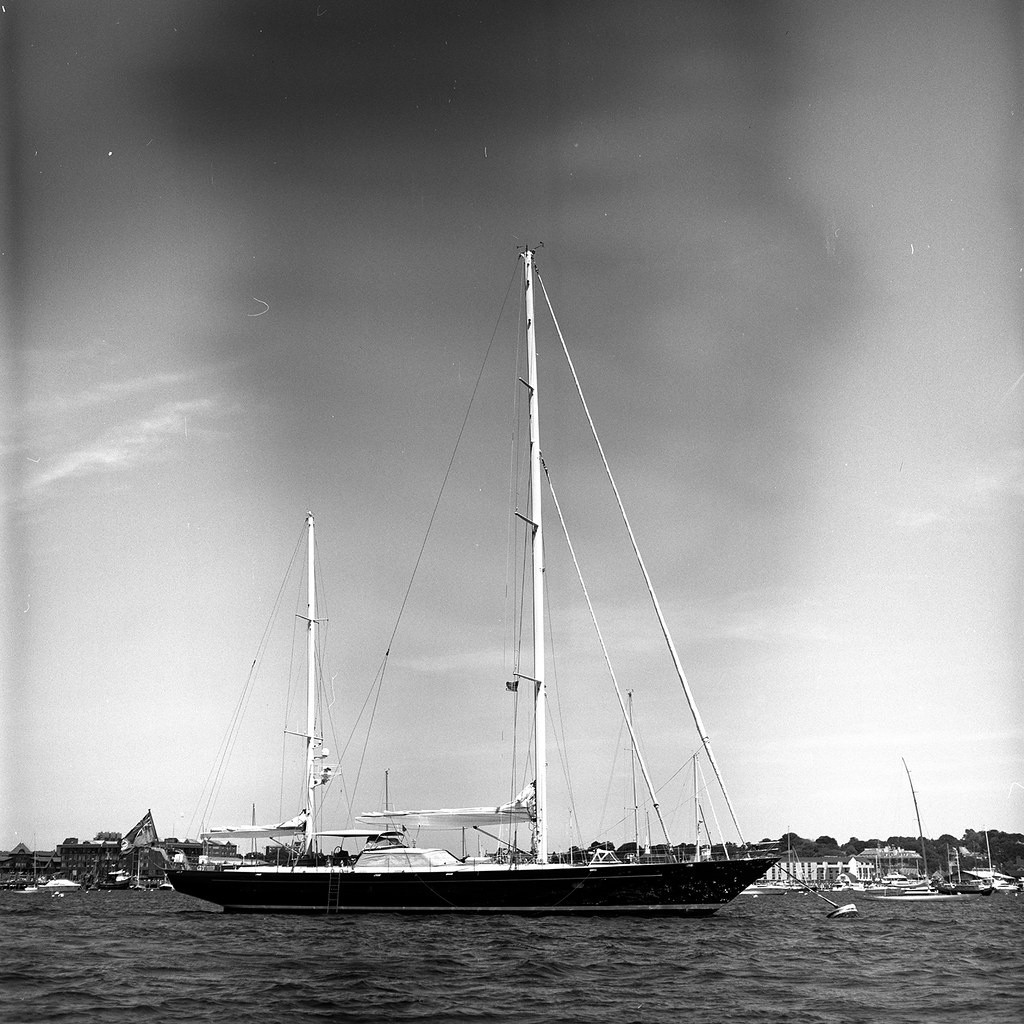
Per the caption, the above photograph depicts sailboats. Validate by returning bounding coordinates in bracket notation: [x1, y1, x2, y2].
[154, 246, 846, 915]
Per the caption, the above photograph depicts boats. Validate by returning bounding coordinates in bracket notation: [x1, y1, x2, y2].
[11, 830, 134, 897]
[857, 764, 1021, 903]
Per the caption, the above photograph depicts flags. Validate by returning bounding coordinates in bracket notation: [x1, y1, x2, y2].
[116, 812, 160, 860]
